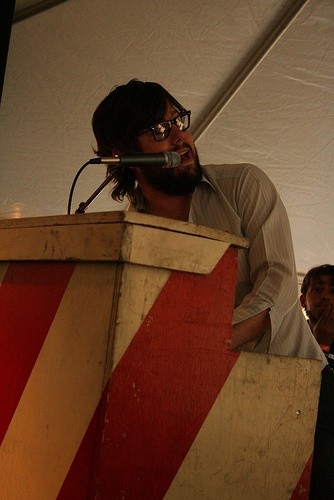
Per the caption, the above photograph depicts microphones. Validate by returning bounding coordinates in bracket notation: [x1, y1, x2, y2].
[89, 150, 181, 169]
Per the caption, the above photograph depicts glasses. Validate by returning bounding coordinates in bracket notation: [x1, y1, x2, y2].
[139, 110, 191, 142]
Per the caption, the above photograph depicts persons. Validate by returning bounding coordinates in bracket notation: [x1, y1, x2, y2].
[90, 79, 334, 500]
